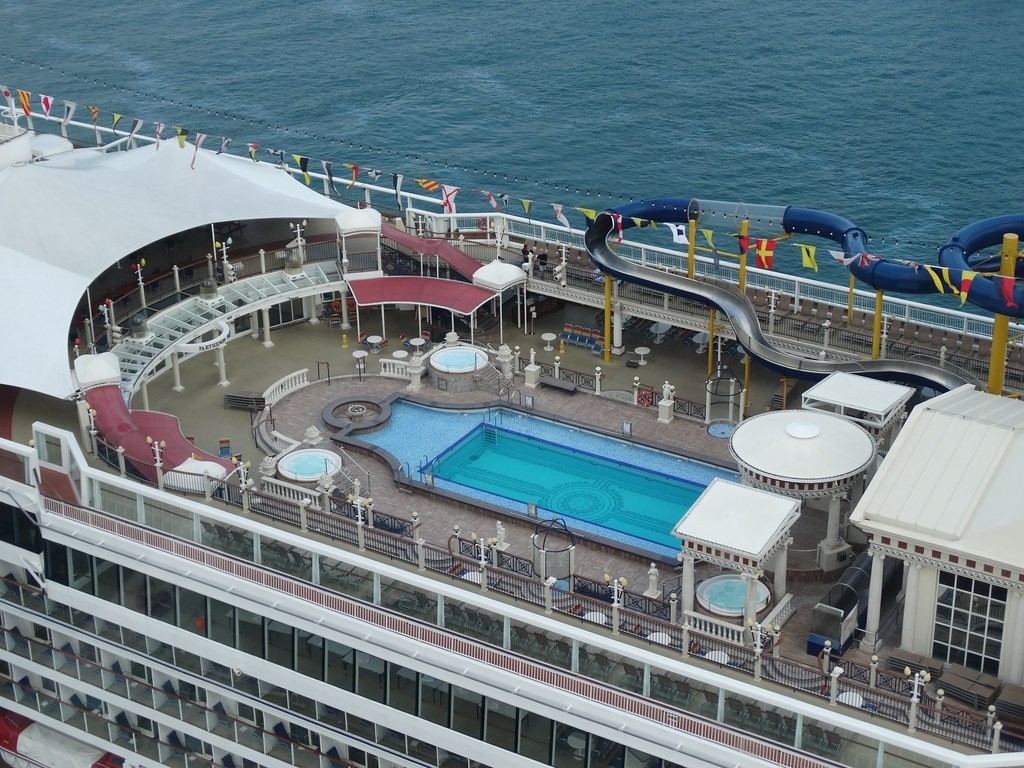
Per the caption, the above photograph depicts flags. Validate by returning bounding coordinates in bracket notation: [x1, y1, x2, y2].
[88, 106, 99, 126]
[321, 161, 340, 195]
[0, 85, 11, 100]
[248, 143, 260, 162]
[703, 228, 818, 271]
[666, 223, 689, 244]
[216, 137, 231, 155]
[830, 249, 1018, 308]
[367, 167, 403, 212]
[176, 128, 188, 148]
[577, 207, 623, 245]
[418, 179, 438, 192]
[191, 133, 205, 169]
[154, 123, 165, 151]
[474, 189, 572, 235]
[64, 101, 76, 125]
[632, 217, 656, 229]
[344, 163, 358, 190]
[17, 90, 32, 116]
[112, 114, 121, 134]
[267, 148, 310, 185]
[443, 186, 459, 213]
[40, 95, 54, 120]
[126, 119, 143, 151]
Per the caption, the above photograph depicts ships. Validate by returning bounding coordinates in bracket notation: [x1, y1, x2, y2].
[0, 107, 1024, 768]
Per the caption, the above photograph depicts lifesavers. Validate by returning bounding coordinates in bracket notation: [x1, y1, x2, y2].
[637, 391, 652, 408]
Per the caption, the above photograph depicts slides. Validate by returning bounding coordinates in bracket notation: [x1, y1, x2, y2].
[584, 197, 1024, 394]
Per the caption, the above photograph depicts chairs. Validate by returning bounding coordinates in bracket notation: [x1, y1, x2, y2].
[675, 639, 707, 656]
[185, 436, 195, 446]
[359, 719, 479, 768]
[885, 320, 1024, 382]
[513, 238, 598, 282]
[557, 642, 854, 768]
[360, 332, 388, 353]
[619, 618, 648, 637]
[400, 331, 430, 353]
[558, 309, 739, 358]
[201, 519, 395, 603]
[631, 265, 739, 312]
[446, 562, 466, 577]
[218, 438, 232, 459]
[323, 299, 357, 331]
[2, 573, 96, 631]
[745, 288, 875, 347]
[388, 583, 572, 673]
[734, 657, 748, 668]
[10, 626, 353, 768]
[569, 603, 585, 617]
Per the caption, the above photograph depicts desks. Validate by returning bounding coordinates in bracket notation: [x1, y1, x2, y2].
[610, 314, 631, 329]
[393, 338, 424, 368]
[836, 692, 864, 708]
[737, 345, 746, 364]
[463, 572, 482, 583]
[541, 333, 556, 351]
[396, 667, 529, 738]
[650, 322, 671, 344]
[583, 612, 606, 624]
[705, 651, 728, 664]
[692, 332, 709, 354]
[352, 336, 382, 368]
[647, 633, 671, 646]
[634, 347, 650, 366]
[225, 607, 390, 689]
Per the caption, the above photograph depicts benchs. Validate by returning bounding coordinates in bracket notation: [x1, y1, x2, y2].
[539, 375, 577, 395]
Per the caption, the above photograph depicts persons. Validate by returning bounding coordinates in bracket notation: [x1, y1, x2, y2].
[522, 244, 547, 280]
